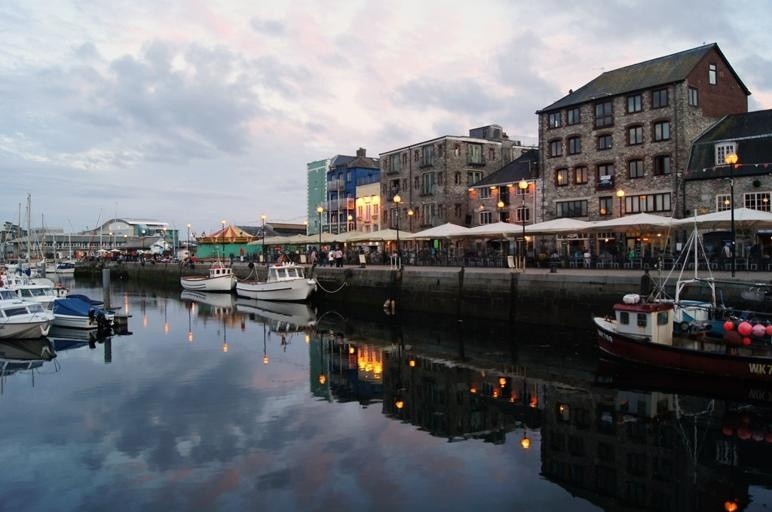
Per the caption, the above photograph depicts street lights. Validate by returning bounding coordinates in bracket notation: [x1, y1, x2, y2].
[725, 151, 738, 277]
[317, 325, 533, 451]
[142, 295, 272, 364]
[108, 211, 268, 268]
[615, 189, 625, 244]
[346, 214, 353, 231]
[518, 178, 529, 272]
[393, 193, 403, 269]
[302, 220, 308, 257]
[316, 206, 324, 265]
[407, 209, 414, 232]
[496, 199, 504, 222]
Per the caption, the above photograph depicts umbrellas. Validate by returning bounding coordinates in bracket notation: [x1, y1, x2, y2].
[468, 219, 524, 256]
[523, 216, 598, 256]
[590, 212, 677, 257]
[678, 204, 772, 259]
[246, 221, 474, 268]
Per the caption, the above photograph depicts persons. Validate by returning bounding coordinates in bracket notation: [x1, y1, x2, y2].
[81, 250, 175, 267]
[583, 249, 592, 264]
[639, 268, 650, 296]
[571, 248, 583, 268]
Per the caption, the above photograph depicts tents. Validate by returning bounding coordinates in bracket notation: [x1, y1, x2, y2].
[196, 223, 262, 259]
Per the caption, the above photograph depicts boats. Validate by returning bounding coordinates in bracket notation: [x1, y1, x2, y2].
[234, 296, 319, 337]
[46, 297, 117, 330]
[1, 286, 57, 339]
[179, 245, 238, 292]
[598, 352, 771, 414]
[180, 288, 238, 316]
[47, 321, 115, 354]
[590, 207, 772, 386]
[0, 337, 59, 395]
[235, 260, 318, 301]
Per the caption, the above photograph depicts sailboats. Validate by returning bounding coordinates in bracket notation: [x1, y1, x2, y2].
[1, 189, 105, 314]
